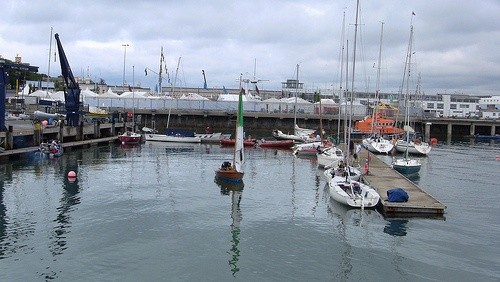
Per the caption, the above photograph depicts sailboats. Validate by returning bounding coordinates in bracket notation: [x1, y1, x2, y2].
[272, 64, 322, 142]
[314, 0, 381, 207]
[395, 25, 431, 154]
[392, 99, 422, 174]
[215, 76, 245, 183]
[362, 22, 394, 153]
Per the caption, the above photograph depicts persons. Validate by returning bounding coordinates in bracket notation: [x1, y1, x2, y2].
[39, 139, 60, 153]
[349, 137, 362, 162]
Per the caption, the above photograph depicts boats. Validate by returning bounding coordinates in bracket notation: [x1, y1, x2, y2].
[118, 131, 142, 144]
[220, 135, 256, 145]
[193, 127, 231, 142]
[142, 127, 201, 142]
[260, 139, 293, 148]
[290, 137, 333, 155]
[39, 140, 64, 157]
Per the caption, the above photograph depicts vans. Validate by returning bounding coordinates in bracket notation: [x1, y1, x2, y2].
[424, 109, 436, 117]
